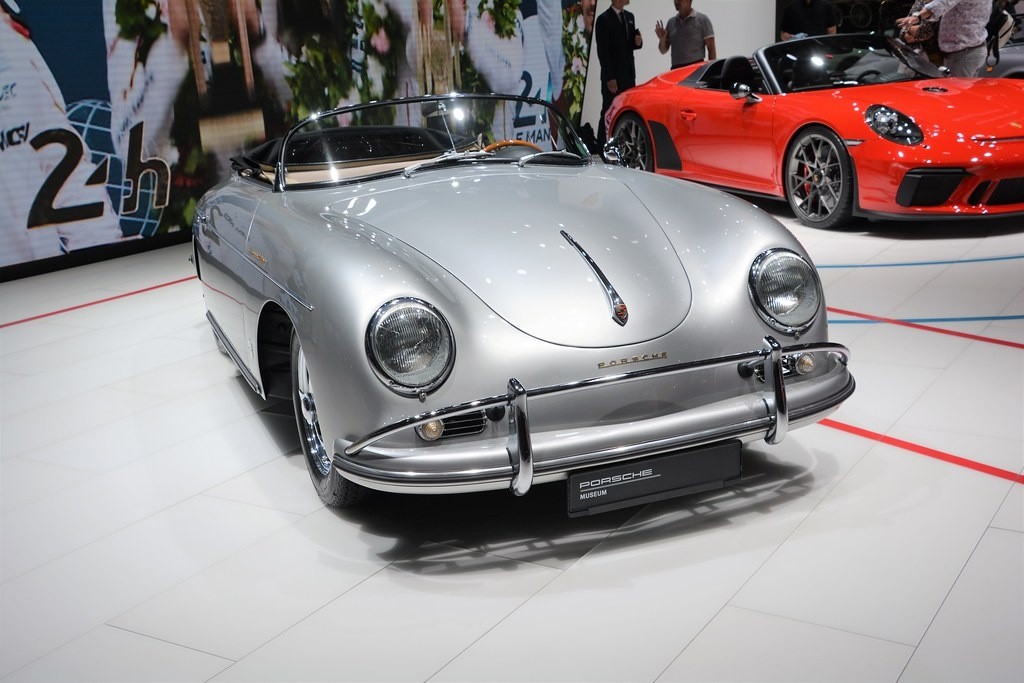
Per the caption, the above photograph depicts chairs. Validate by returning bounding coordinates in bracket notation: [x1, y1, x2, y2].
[791, 52, 827, 81]
[721, 55, 754, 76]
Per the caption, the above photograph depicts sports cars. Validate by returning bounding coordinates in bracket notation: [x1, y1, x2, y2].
[604, 29, 1024, 229]
[827, 10, 1024, 86]
[188, 93, 857, 511]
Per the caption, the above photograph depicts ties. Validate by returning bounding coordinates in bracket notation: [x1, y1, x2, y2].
[619, 11, 628, 40]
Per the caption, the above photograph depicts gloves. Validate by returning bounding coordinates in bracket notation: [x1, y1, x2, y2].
[791, 33, 808, 40]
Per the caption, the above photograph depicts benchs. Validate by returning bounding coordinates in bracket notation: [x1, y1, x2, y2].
[267, 125, 467, 165]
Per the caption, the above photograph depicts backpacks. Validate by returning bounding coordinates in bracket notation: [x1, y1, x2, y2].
[986, 0, 1015, 68]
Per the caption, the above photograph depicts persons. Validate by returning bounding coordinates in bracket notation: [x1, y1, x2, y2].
[780, 0, 840, 42]
[655, 0, 716, 71]
[595, 0, 642, 155]
[896, 0, 992, 79]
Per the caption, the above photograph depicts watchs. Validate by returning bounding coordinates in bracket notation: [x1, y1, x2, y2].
[912, 11, 922, 23]
